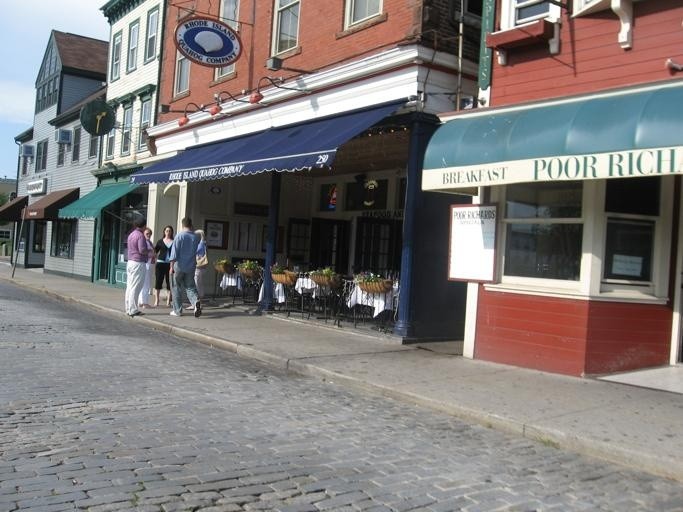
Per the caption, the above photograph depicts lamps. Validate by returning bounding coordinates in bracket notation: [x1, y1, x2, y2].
[178, 77, 311, 127]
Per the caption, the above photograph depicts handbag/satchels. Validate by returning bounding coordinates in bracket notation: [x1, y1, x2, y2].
[195, 244, 208, 269]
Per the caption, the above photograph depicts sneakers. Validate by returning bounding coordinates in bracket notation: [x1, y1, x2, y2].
[143, 304, 155, 309]
[185, 305, 194, 310]
[170, 310, 178, 317]
[194, 302, 200, 317]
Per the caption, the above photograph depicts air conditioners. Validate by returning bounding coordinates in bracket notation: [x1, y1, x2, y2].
[18, 145, 33, 157]
[53, 129, 71, 144]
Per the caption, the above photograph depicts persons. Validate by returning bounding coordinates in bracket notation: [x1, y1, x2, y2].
[138, 228, 156, 308]
[125, 216, 148, 316]
[170, 216, 201, 317]
[151, 225, 174, 307]
[185, 229, 208, 310]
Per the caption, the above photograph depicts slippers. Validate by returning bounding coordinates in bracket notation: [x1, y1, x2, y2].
[130, 312, 145, 316]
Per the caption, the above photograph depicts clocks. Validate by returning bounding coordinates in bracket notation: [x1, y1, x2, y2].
[79, 98, 116, 137]
